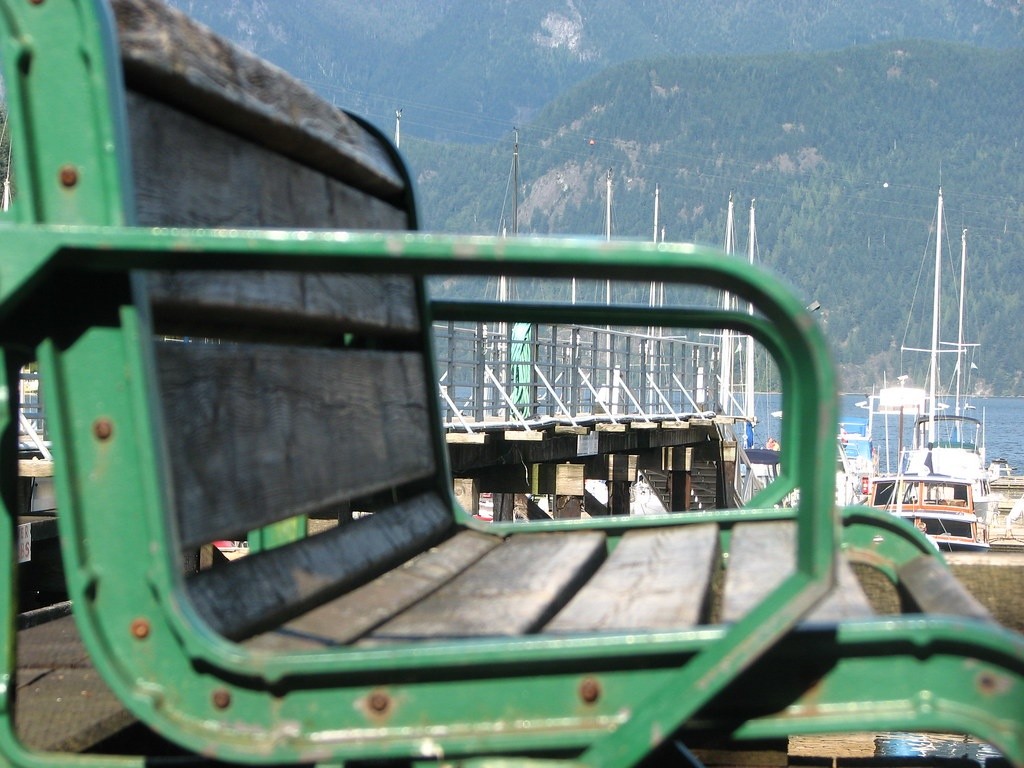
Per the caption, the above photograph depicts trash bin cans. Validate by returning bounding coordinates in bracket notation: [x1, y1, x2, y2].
[19, 439, 51, 516]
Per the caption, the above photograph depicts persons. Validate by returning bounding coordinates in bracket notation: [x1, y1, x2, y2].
[766, 437, 780, 480]
[918, 522, 940, 553]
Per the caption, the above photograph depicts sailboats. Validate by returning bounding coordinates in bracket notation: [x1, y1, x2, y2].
[439, 128, 1024, 551]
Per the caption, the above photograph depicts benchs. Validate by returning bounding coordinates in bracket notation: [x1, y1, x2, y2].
[0, 0, 1024, 768]
